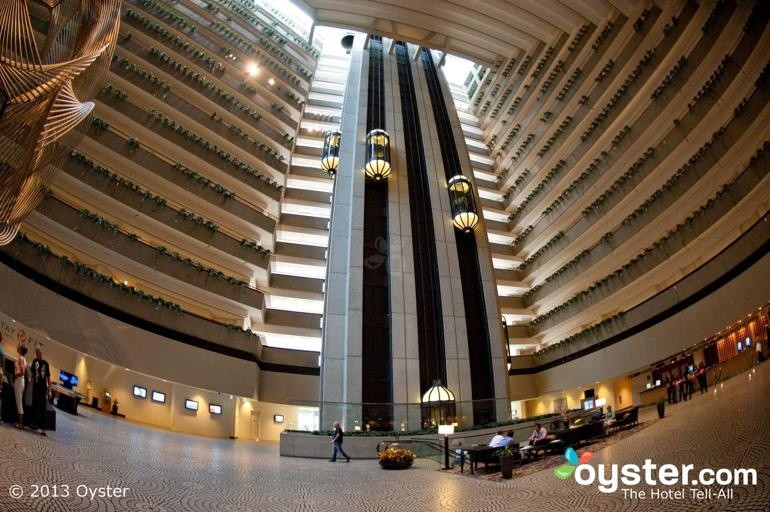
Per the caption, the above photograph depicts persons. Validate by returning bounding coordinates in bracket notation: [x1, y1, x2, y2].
[604, 405, 617, 435]
[451, 441, 466, 467]
[662, 361, 710, 405]
[499, 430, 516, 447]
[13, 341, 28, 429]
[754, 331, 763, 365]
[0, 332, 7, 426]
[527, 423, 550, 455]
[329, 420, 351, 463]
[489, 430, 504, 447]
[28, 343, 52, 434]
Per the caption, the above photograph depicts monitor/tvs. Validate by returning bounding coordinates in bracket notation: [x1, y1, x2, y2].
[688, 364, 694, 374]
[581, 397, 595, 410]
[745, 337, 750, 346]
[185, 398, 198, 410]
[151, 390, 165, 403]
[209, 403, 222, 415]
[132, 385, 147, 399]
[737, 341, 742, 351]
[59, 369, 78, 390]
[274, 415, 284, 422]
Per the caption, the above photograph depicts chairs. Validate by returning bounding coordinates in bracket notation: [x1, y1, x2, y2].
[450, 402, 637, 479]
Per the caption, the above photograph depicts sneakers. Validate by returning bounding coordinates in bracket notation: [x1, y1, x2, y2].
[328, 459, 336, 462]
[345, 457, 351, 462]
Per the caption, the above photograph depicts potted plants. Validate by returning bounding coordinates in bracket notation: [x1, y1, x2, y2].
[375, 447, 419, 470]
[653, 393, 670, 419]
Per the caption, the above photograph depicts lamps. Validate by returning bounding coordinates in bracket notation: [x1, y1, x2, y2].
[436, 423, 455, 470]
